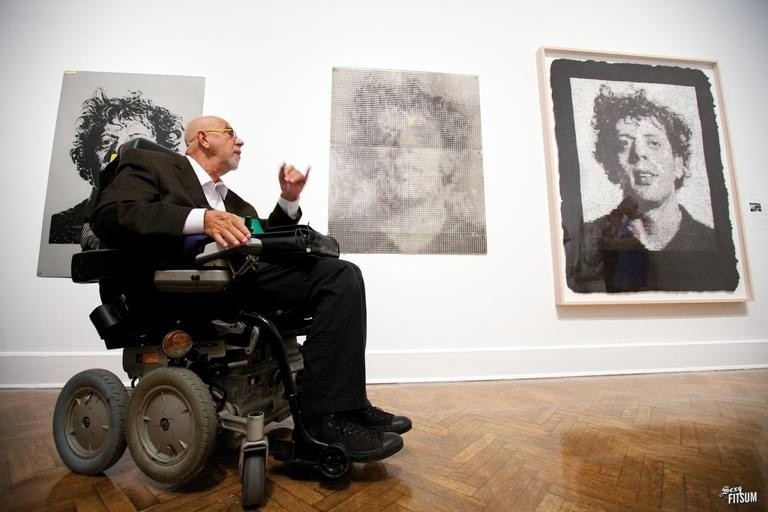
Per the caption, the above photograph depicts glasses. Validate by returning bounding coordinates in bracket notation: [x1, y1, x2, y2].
[190, 127, 237, 145]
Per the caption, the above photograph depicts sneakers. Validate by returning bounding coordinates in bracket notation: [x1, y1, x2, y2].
[336, 407, 413, 436]
[291, 407, 404, 463]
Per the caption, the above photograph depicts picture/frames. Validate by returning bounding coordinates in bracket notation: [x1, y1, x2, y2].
[536, 47, 755, 307]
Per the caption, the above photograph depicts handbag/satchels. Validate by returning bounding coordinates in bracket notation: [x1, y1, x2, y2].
[233, 215, 341, 274]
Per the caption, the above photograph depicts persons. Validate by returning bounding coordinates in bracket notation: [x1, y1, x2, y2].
[49, 93, 183, 244]
[581, 92, 716, 285]
[88, 116, 412, 462]
[329, 75, 487, 254]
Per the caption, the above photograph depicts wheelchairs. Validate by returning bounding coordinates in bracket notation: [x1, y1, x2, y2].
[53, 138, 351, 508]
